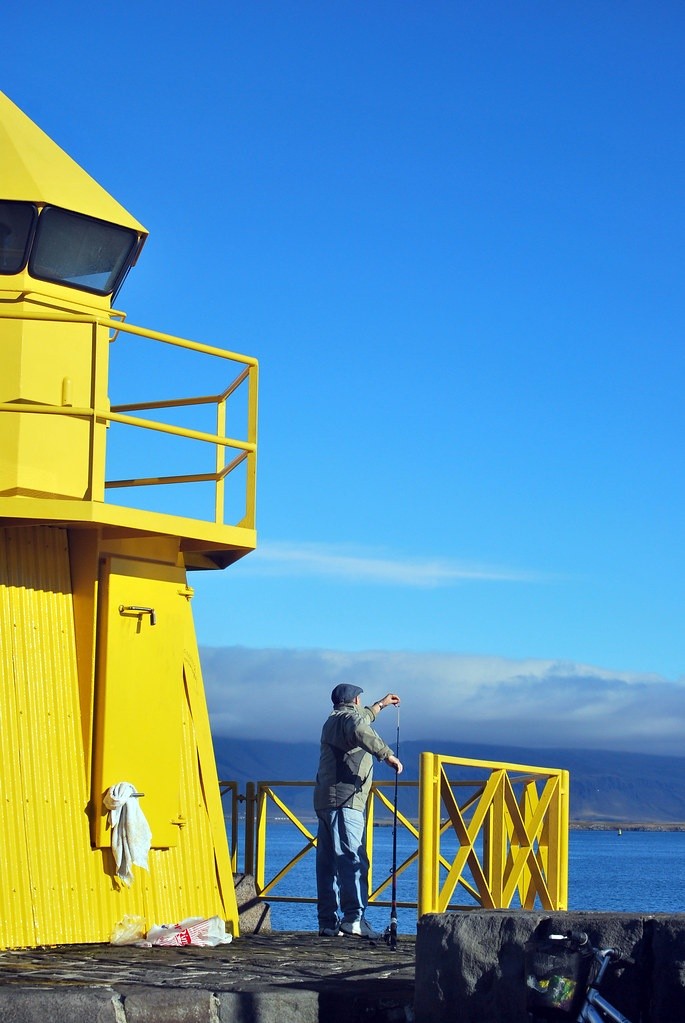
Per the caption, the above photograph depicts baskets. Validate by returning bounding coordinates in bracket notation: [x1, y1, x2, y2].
[525, 940, 583, 1011]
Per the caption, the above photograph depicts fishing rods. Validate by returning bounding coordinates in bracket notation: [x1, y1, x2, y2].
[384, 701, 401, 952]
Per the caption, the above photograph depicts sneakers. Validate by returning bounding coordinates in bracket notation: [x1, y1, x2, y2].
[339, 920, 381, 939]
[319, 927, 340, 937]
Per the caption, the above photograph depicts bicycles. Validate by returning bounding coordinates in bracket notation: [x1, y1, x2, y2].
[524, 929, 638, 1023]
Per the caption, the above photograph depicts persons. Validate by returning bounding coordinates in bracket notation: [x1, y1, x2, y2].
[313, 684, 403, 939]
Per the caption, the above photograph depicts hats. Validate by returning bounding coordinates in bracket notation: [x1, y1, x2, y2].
[330, 683, 363, 704]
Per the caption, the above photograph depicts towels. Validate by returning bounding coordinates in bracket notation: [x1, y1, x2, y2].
[103, 782, 153, 886]
